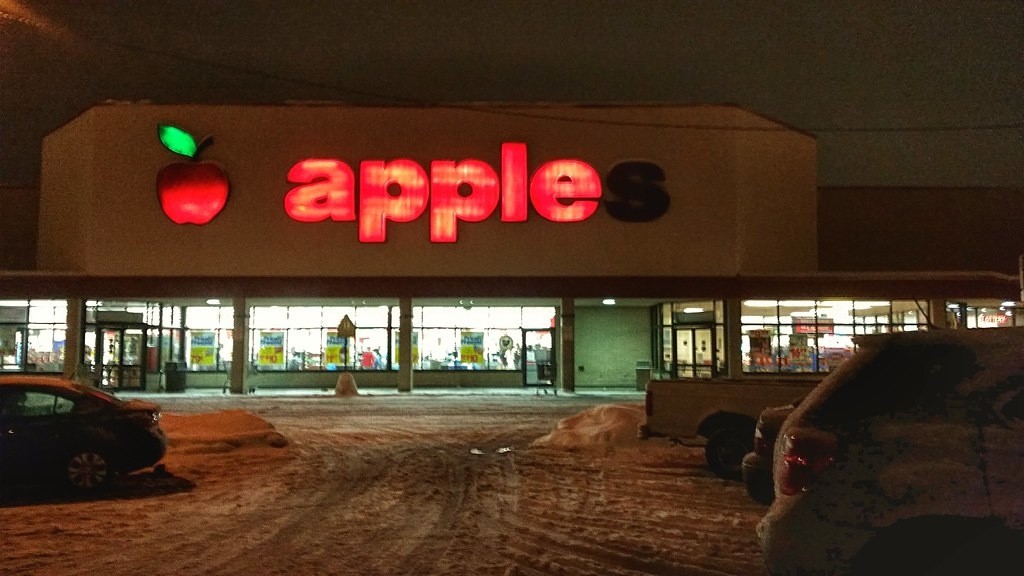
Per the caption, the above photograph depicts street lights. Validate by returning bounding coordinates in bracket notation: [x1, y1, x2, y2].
[336, 314, 358, 396]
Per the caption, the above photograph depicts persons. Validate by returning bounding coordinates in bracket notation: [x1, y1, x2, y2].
[362, 347, 380, 369]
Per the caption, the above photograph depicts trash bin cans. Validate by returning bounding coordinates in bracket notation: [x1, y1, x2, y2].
[164, 361, 187, 393]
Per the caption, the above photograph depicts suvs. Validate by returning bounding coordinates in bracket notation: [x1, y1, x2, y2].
[756, 326, 1024, 576]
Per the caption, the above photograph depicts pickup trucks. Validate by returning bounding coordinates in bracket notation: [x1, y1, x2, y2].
[644, 370, 832, 478]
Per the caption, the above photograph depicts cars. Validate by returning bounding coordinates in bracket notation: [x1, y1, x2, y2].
[742, 393, 815, 492]
[0, 376, 166, 494]
